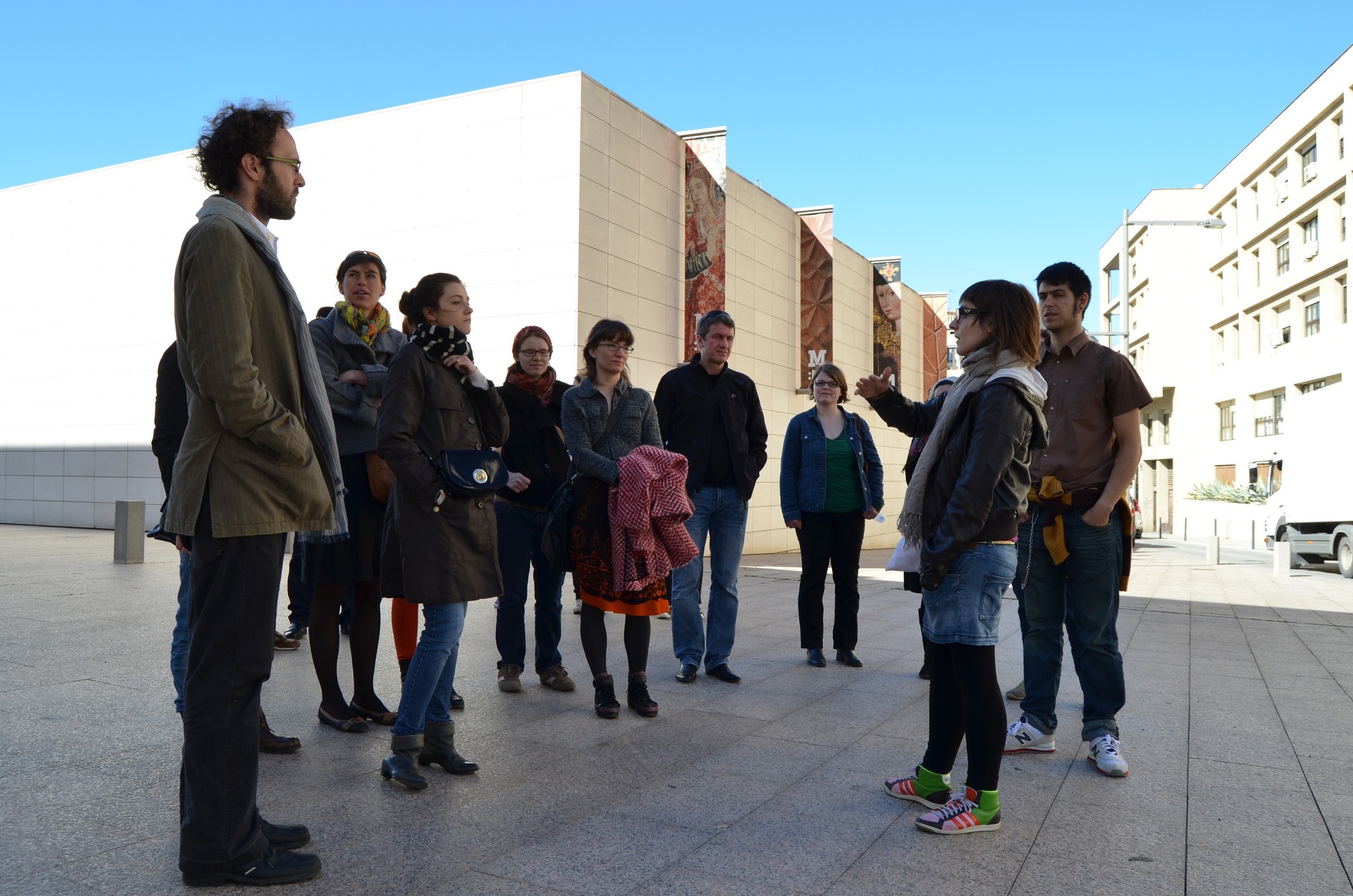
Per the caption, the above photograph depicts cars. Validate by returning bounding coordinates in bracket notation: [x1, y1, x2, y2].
[1126, 482, 1143, 548]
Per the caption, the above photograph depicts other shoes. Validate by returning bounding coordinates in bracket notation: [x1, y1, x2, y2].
[573, 599, 583, 614]
[494, 597, 499, 607]
[656, 606, 672, 619]
[699, 607, 704, 619]
[182, 619, 576, 790]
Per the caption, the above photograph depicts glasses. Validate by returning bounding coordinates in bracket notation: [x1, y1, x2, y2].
[260, 156, 301, 174]
[598, 343, 634, 354]
[345, 251, 382, 261]
[956, 308, 985, 322]
[813, 380, 841, 387]
[518, 349, 550, 357]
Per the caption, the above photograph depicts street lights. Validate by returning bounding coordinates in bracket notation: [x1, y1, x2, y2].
[1081, 208, 1227, 360]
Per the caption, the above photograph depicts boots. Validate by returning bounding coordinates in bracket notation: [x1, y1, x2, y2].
[918, 609, 931, 680]
[592, 674, 621, 718]
[626, 675, 658, 717]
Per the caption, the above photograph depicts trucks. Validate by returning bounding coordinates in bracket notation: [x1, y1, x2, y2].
[1263, 376, 1353, 578]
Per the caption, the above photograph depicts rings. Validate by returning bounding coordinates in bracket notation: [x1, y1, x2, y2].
[457, 357, 461, 361]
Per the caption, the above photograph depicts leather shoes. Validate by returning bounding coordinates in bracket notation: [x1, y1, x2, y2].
[807, 648, 826, 668]
[836, 649, 863, 667]
[676, 663, 697, 683]
[705, 663, 741, 683]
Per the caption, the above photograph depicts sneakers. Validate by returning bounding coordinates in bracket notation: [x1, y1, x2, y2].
[914, 782, 1001, 834]
[1006, 677, 1027, 699]
[1087, 735, 1129, 778]
[1002, 716, 1054, 753]
[883, 762, 952, 810]
[258, 817, 310, 849]
[182, 845, 321, 886]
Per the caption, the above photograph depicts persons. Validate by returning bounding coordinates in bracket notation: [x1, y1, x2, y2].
[901, 376, 959, 679]
[779, 363, 884, 666]
[853, 279, 1050, 836]
[160, 101, 349, 884]
[274, 251, 581, 791]
[1001, 261, 1154, 777]
[151, 340, 302, 754]
[562, 308, 768, 718]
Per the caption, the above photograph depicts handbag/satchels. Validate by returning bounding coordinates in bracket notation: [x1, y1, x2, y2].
[367, 452, 396, 503]
[438, 448, 509, 496]
[885, 535, 921, 573]
